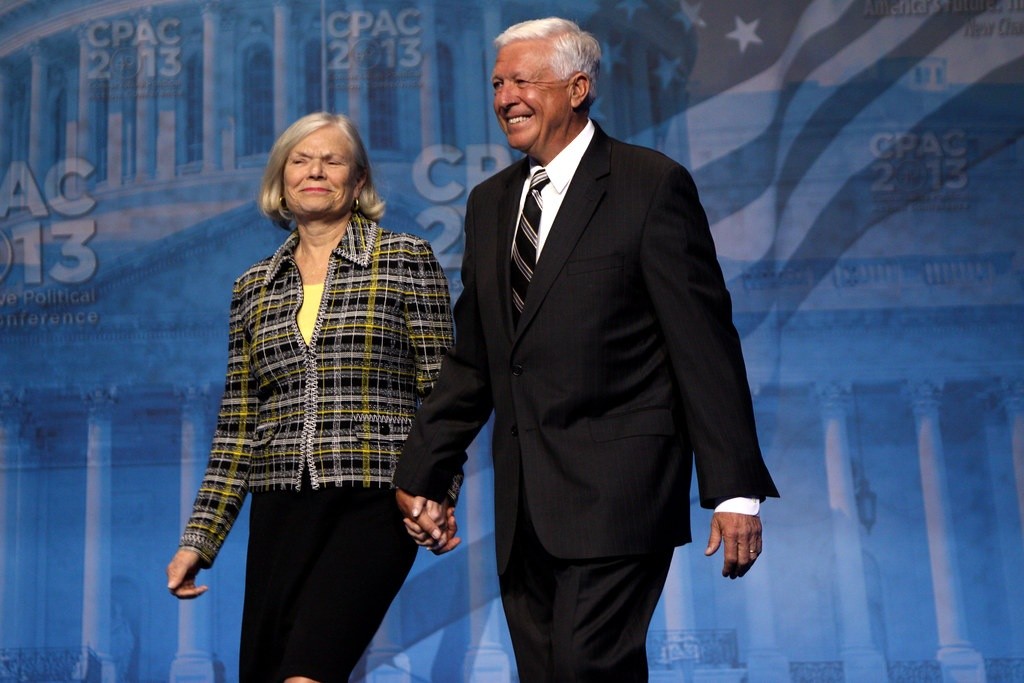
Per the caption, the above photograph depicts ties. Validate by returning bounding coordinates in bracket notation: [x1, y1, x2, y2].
[510, 168, 550, 333]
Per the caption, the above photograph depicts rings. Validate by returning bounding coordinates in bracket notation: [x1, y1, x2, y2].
[750, 549, 756, 553]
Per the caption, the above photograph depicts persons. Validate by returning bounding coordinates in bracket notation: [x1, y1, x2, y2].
[165, 112, 453, 683]
[392, 18, 783, 683]
